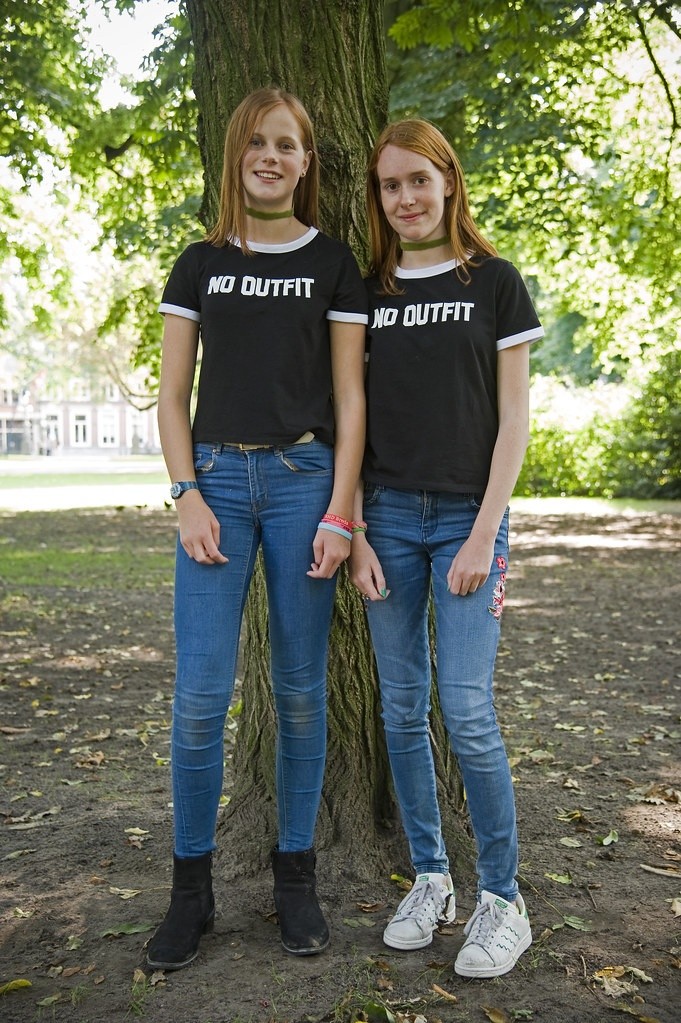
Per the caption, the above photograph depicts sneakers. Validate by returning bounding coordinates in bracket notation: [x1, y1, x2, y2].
[454, 890, 533, 978]
[383, 873, 456, 950]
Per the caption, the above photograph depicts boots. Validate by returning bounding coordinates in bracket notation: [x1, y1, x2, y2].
[270, 844, 330, 955]
[147, 850, 214, 969]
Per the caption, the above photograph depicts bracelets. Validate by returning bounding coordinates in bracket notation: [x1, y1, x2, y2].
[317, 513, 352, 540]
[352, 520, 368, 533]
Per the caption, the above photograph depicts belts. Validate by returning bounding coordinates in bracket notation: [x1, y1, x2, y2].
[222, 430, 314, 453]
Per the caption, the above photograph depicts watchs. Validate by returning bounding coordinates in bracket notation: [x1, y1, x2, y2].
[170, 481, 199, 500]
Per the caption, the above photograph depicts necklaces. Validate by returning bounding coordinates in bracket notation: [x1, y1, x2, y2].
[241, 203, 297, 220]
[397, 236, 450, 252]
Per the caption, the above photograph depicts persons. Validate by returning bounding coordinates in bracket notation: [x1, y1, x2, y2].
[143, 86, 369, 969]
[346, 119, 545, 978]
